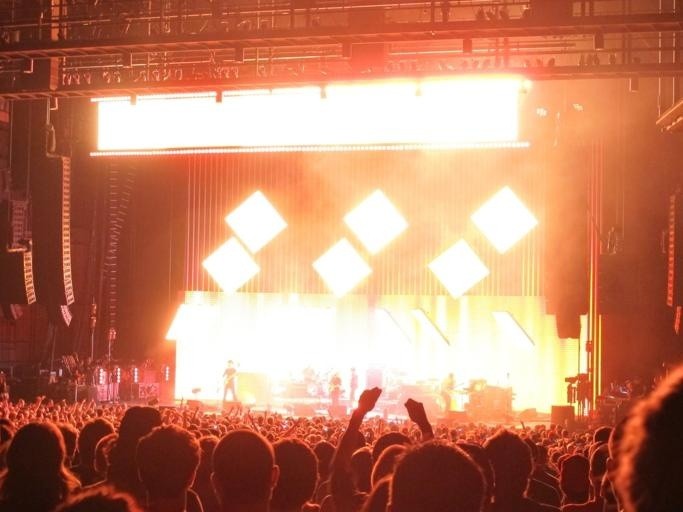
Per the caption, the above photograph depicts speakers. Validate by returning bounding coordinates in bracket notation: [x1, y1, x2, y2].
[539, 162, 590, 341]
[31, 154, 76, 328]
[4, 247, 37, 305]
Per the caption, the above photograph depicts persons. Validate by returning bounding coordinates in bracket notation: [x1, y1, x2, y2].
[440, 372, 454, 415]
[329, 373, 340, 405]
[222, 364, 237, 403]
[349, 368, 358, 405]
[1, 364, 681, 512]
